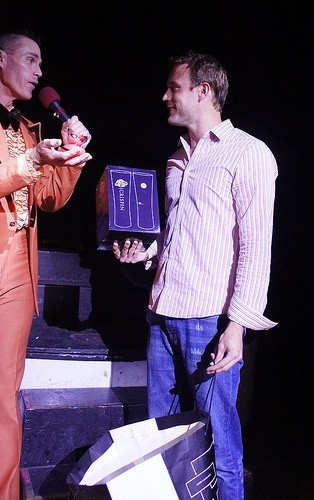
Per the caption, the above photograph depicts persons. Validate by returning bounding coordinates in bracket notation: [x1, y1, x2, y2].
[0, 34, 92, 500]
[112, 51, 281, 499]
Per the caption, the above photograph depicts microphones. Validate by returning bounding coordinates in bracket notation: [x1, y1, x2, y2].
[38, 87, 88, 144]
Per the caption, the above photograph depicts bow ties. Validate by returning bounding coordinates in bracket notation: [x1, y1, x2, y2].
[0, 102, 22, 130]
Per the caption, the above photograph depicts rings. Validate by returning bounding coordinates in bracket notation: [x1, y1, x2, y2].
[70, 132, 78, 139]
[78, 134, 87, 143]
